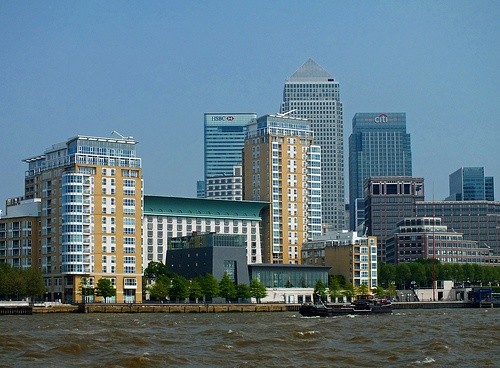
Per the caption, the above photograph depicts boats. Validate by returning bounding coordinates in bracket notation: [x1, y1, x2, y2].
[298, 291, 392, 316]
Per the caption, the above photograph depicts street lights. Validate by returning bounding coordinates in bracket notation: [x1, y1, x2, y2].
[410, 280, 416, 302]
